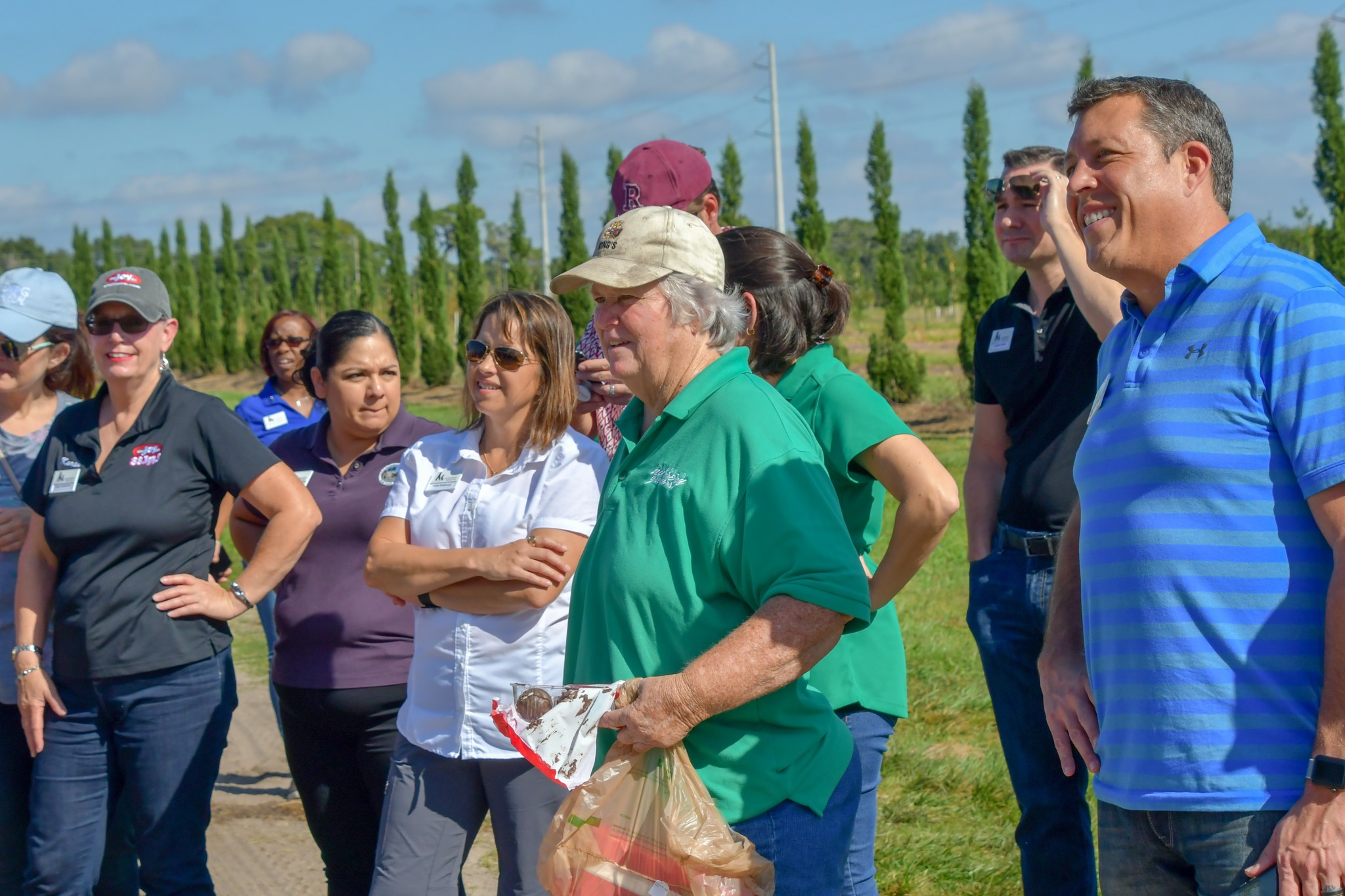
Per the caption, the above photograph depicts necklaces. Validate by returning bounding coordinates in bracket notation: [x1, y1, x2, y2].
[481, 436, 517, 477]
[274, 385, 311, 407]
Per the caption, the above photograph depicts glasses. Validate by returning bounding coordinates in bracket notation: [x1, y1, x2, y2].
[464, 339, 542, 371]
[262, 337, 312, 350]
[84, 316, 150, 335]
[984, 174, 1049, 205]
[0, 339, 55, 360]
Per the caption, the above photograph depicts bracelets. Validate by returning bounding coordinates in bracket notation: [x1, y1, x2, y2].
[14, 666, 45, 685]
[230, 582, 254, 607]
[417, 593, 439, 608]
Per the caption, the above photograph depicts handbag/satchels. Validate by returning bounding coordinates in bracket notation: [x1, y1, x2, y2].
[536, 735, 777, 896]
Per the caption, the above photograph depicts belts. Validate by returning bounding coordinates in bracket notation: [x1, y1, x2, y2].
[993, 524, 1061, 558]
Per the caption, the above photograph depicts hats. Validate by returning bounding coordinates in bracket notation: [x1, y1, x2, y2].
[0, 266, 78, 343]
[84, 266, 173, 324]
[550, 206, 727, 296]
[610, 140, 713, 220]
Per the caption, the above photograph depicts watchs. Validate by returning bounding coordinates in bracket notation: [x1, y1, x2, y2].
[11, 644, 43, 661]
[1305, 755, 1345, 792]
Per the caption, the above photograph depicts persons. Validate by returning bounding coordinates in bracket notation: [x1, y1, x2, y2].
[715, 225, 963, 896]
[963, 145, 1127, 896]
[1038, 78, 1345, 896]
[10, 265, 323, 896]
[364, 291, 611, 896]
[548, 205, 872, 895]
[569, 140, 738, 461]
[229, 309, 466, 896]
[210, 310, 329, 799]
[0, 268, 94, 896]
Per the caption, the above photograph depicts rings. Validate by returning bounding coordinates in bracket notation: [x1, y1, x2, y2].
[608, 383, 615, 399]
[1320, 889, 1345, 896]
[1056, 176, 1061, 179]
[528, 536, 536, 548]
